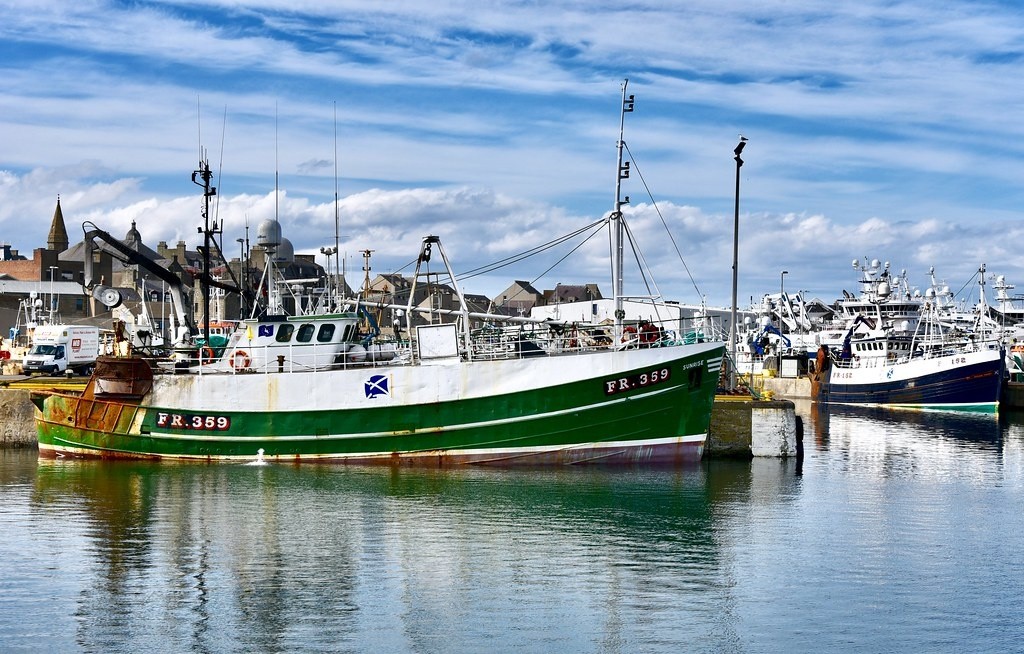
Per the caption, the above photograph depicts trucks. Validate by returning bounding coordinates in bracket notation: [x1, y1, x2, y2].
[22, 325, 99, 376]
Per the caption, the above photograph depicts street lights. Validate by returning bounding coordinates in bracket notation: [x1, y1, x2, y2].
[779, 271, 789, 377]
[798, 288, 810, 346]
[236, 238, 245, 319]
[729, 142, 749, 389]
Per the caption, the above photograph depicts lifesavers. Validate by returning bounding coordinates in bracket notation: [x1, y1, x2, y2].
[621, 327, 638, 343]
[640, 324, 657, 343]
[230, 351, 251, 371]
[197, 346, 213, 364]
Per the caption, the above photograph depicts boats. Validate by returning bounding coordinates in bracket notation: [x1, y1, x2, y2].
[27, 78, 726, 465]
[693, 253, 1023, 420]
